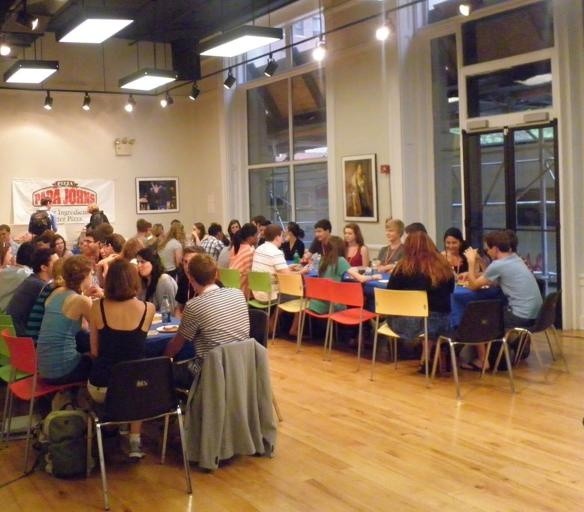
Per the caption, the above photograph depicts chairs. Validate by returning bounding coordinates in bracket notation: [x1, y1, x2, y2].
[490, 290, 566, 383]
[429, 299, 516, 401]
[299, 276, 339, 361]
[270, 272, 312, 350]
[326, 278, 394, 371]
[369, 285, 442, 388]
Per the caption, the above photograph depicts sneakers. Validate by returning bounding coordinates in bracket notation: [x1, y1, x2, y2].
[118, 432, 147, 458]
[416, 364, 439, 375]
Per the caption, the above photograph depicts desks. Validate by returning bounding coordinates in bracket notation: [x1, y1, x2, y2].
[288, 264, 501, 330]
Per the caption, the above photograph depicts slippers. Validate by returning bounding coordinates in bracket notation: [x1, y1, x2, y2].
[459, 360, 489, 372]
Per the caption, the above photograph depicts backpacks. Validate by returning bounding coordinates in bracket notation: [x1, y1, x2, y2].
[27, 209, 52, 235]
[33, 402, 98, 481]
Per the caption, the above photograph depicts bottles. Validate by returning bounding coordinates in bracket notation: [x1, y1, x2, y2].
[160, 295, 170, 325]
[292, 250, 299, 264]
[371, 258, 378, 275]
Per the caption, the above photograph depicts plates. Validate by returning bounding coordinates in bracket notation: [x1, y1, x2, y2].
[155, 325, 179, 333]
[378, 280, 389, 284]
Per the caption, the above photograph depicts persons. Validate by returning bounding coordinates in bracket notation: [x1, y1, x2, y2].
[139, 182, 172, 210]
[82, 203, 110, 228]
[28, 196, 57, 235]
[506, 229, 534, 275]
[464, 228, 543, 370]
[228, 217, 484, 374]
[0, 217, 250, 427]
[86, 257, 160, 460]
[349, 162, 373, 215]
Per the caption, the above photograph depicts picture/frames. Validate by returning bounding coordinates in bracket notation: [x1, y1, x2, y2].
[135, 177, 180, 214]
[341, 155, 378, 224]
